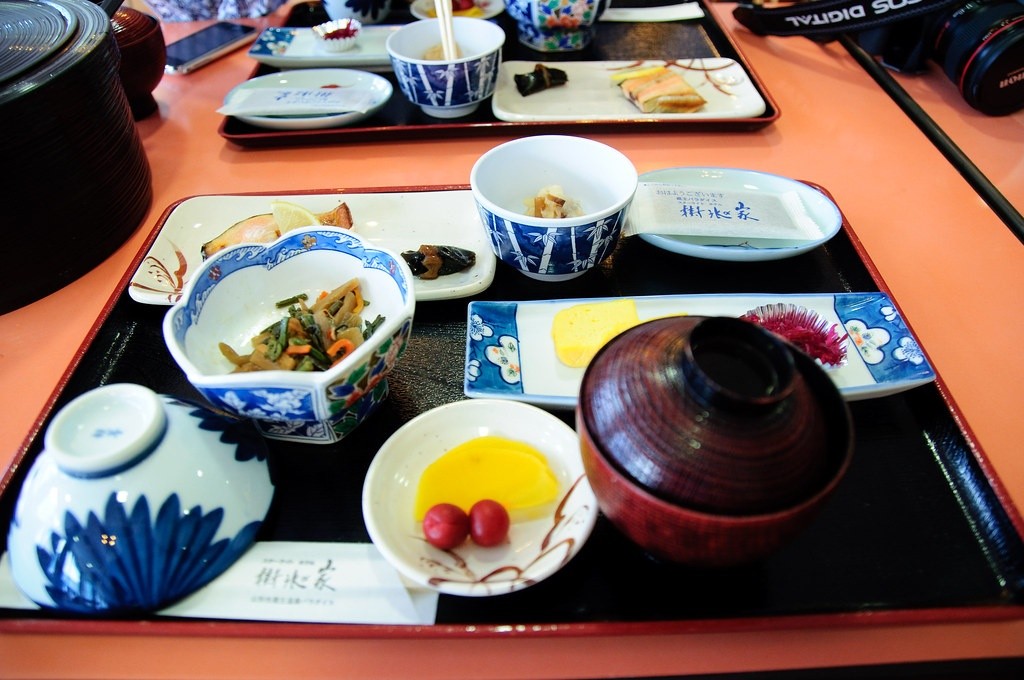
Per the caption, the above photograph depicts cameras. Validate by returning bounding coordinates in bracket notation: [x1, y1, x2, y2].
[843, 0, 1024, 119]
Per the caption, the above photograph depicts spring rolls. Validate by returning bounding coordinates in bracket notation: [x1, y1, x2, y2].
[614, 68, 703, 113]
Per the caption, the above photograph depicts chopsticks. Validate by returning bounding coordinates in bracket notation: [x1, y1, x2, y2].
[434, 0, 458, 60]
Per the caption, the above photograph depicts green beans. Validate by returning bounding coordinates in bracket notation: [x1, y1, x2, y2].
[264, 293, 385, 374]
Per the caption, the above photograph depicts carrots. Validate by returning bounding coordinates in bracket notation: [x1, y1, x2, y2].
[326, 340, 354, 367]
[285, 344, 310, 354]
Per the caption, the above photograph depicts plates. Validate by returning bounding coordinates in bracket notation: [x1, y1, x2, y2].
[464, 292, 937, 410]
[224, 68, 393, 129]
[129, 190, 496, 305]
[361, 399, 598, 596]
[638, 167, 842, 261]
[246, 24, 405, 72]
[411, 0, 505, 20]
[492, 58, 766, 122]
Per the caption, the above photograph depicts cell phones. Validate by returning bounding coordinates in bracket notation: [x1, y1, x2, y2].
[164, 21, 261, 74]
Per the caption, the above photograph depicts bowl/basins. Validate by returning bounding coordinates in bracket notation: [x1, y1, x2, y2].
[7, 384, 276, 616]
[163, 226, 416, 444]
[386, 16, 506, 118]
[470, 135, 638, 281]
[575, 315, 856, 563]
[503, 0, 611, 51]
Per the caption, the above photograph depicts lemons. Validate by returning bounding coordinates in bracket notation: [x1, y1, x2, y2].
[271, 202, 321, 238]
